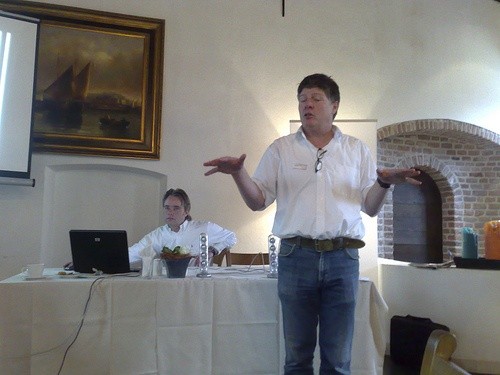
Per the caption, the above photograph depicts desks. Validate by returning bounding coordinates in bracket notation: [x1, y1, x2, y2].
[0, 264, 389, 375]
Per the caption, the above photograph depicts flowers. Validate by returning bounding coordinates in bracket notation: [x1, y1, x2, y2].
[163, 246, 190, 255]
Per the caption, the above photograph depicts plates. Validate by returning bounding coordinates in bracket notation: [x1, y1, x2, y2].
[23, 275, 49, 278]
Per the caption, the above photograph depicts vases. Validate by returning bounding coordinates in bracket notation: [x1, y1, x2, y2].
[165, 256, 195, 278]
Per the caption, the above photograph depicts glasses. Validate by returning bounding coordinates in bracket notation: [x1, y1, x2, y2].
[314, 148, 327, 173]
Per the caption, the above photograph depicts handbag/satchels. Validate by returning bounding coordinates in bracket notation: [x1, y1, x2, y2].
[390, 314, 450, 371]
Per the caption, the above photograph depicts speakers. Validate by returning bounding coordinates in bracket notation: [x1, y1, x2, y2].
[197, 232, 212, 278]
[269, 234, 278, 278]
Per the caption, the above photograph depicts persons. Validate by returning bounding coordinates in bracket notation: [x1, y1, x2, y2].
[203, 74, 422, 375]
[129, 188, 237, 269]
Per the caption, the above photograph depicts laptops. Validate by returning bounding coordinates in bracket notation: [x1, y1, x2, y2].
[69, 230, 142, 273]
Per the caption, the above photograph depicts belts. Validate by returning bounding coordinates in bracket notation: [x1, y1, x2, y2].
[285, 235, 365, 253]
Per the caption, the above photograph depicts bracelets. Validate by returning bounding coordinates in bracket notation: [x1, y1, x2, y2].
[208, 246, 218, 257]
[377, 177, 390, 188]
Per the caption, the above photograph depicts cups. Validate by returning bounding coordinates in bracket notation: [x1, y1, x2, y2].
[20, 263, 44, 276]
[142, 257, 162, 277]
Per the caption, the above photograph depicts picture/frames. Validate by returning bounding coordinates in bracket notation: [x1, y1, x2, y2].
[0, 0, 165, 160]
